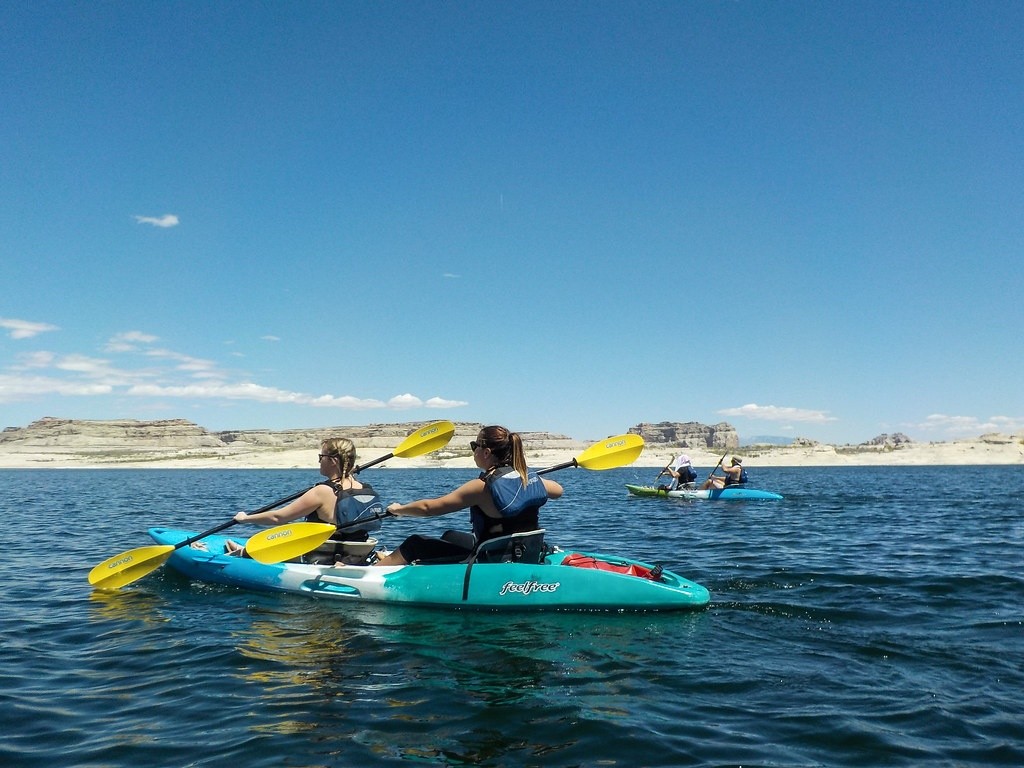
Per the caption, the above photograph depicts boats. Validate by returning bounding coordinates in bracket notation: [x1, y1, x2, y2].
[623, 482, 784, 501]
[149, 524, 712, 612]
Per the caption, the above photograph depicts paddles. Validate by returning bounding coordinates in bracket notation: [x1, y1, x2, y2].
[652, 454, 676, 486]
[244, 432, 645, 567]
[87, 419, 457, 591]
[709, 449, 730, 479]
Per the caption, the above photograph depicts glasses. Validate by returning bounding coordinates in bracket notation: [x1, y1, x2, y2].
[318, 454, 334, 462]
[470, 441, 483, 451]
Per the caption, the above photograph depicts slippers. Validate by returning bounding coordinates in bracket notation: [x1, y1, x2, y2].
[188, 537, 206, 551]
[225, 539, 239, 553]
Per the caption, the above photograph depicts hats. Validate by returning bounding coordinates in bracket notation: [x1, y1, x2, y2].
[731, 456, 743, 466]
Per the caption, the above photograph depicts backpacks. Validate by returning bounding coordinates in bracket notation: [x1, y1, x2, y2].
[732, 466, 748, 483]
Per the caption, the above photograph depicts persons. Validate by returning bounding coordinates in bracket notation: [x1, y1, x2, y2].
[189, 437, 378, 559]
[373, 426, 563, 566]
[698, 455, 742, 490]
[642, 455, 692, 489]
